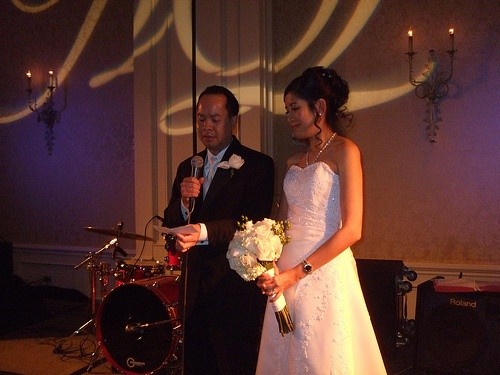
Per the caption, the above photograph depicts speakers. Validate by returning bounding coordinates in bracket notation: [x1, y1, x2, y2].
[356, 258, 403, 351]
[413, 277, 500, 375]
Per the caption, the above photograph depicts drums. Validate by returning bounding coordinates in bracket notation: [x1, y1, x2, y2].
[115, 259, 166, 288]
[167, 249, 183, 272]
[95, 273, 187, 375]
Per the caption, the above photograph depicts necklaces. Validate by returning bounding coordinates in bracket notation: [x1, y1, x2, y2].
[306, 130, 339, 167]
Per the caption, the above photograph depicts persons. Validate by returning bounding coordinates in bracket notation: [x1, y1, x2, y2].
[258, 65, 387, 375]
[164, 85, 273, 375]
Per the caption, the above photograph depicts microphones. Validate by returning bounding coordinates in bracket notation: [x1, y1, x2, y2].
[74, 238, 118, 270]
[188, 155, 203, 213]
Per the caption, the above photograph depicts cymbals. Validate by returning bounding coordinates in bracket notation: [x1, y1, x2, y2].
[84, 227, 154, 241]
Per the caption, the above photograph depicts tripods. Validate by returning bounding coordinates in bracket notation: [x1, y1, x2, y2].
[54, 256, 102, 355]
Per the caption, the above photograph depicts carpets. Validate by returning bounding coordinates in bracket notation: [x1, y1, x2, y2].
[0, 285, 100, 341]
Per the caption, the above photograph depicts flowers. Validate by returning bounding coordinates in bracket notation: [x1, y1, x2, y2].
[217, 154, 245, 177]
[226, 215, 295, 338]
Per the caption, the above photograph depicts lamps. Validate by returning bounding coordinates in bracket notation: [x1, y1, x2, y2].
[405, 27, 459, 144]
[27, 69, 58, 157]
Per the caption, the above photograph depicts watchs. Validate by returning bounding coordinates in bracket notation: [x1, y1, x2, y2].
[302, 260, 312, 275]
